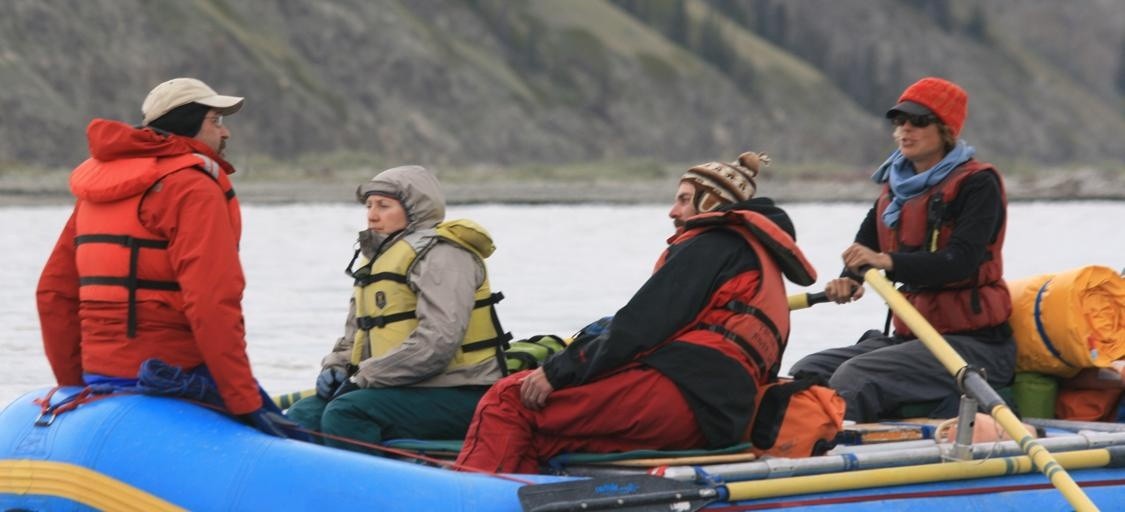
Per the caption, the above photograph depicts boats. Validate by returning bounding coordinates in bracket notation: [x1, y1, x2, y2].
[1, 372, 1125, 512]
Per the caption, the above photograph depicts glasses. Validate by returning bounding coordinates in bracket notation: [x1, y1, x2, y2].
[890, 112, 940, 129]
[195, 113, 225, 128]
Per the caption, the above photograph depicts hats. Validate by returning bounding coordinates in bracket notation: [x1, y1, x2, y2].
[677, 149, 773, 216]
[139, 75, 247, 129]
[883, 74, 969, 138]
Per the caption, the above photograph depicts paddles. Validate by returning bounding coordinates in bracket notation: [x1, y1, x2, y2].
[518, 442, 1125, 512]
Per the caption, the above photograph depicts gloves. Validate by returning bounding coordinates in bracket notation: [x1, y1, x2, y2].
[249, 407, 301, 438]
[315, 363, 361, 403]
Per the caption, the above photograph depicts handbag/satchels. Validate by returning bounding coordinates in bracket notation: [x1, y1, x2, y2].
[741, 368, 850, 460]
[499, 333, 568, 377]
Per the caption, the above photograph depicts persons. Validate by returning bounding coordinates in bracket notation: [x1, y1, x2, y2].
[453, 151, 819, 480]
[283, 162, 511, 456]
[33, 76, 307, 440]
[785, 74, 1017, 423]
